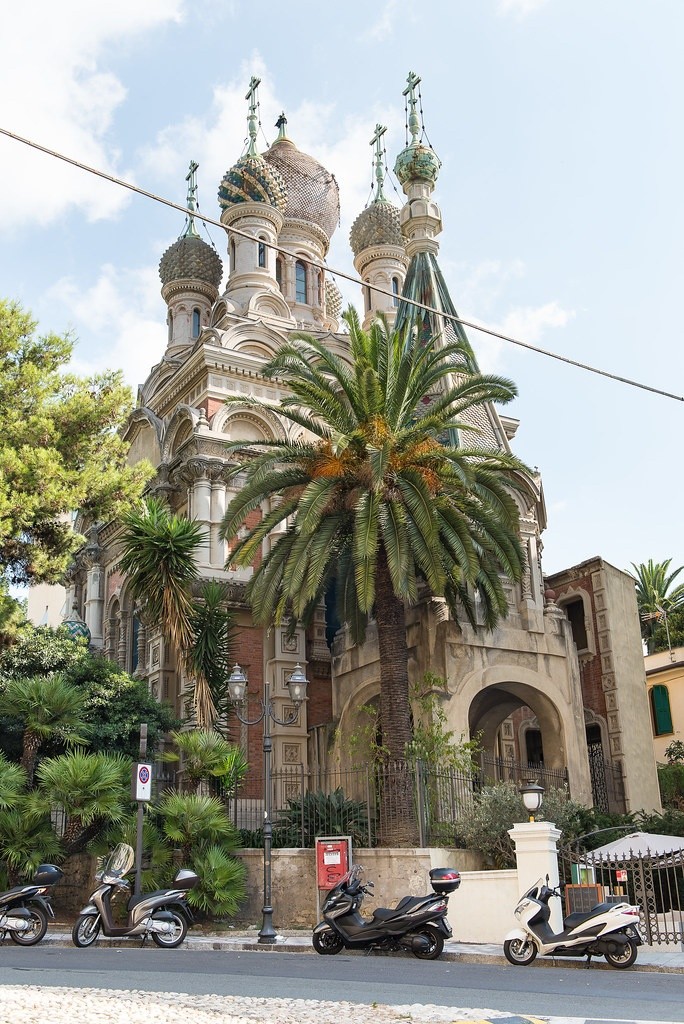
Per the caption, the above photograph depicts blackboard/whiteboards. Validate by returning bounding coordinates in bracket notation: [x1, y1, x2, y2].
[566, 883, 602, 918]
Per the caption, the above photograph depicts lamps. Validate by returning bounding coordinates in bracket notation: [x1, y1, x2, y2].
[519, 779, 546, 820]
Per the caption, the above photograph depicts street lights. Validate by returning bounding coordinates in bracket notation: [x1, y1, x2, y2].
[228, 661, 311, 943]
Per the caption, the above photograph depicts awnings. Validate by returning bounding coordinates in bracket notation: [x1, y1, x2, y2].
[578, 832, 684, 861]
[652, 850, 684, 866]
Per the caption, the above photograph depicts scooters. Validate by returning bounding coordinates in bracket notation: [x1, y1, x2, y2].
[71, 842, 200, 949]
[0, 863, 64, 946]
[503, 873, 644, 971]
[312, 865, 461, 960]
